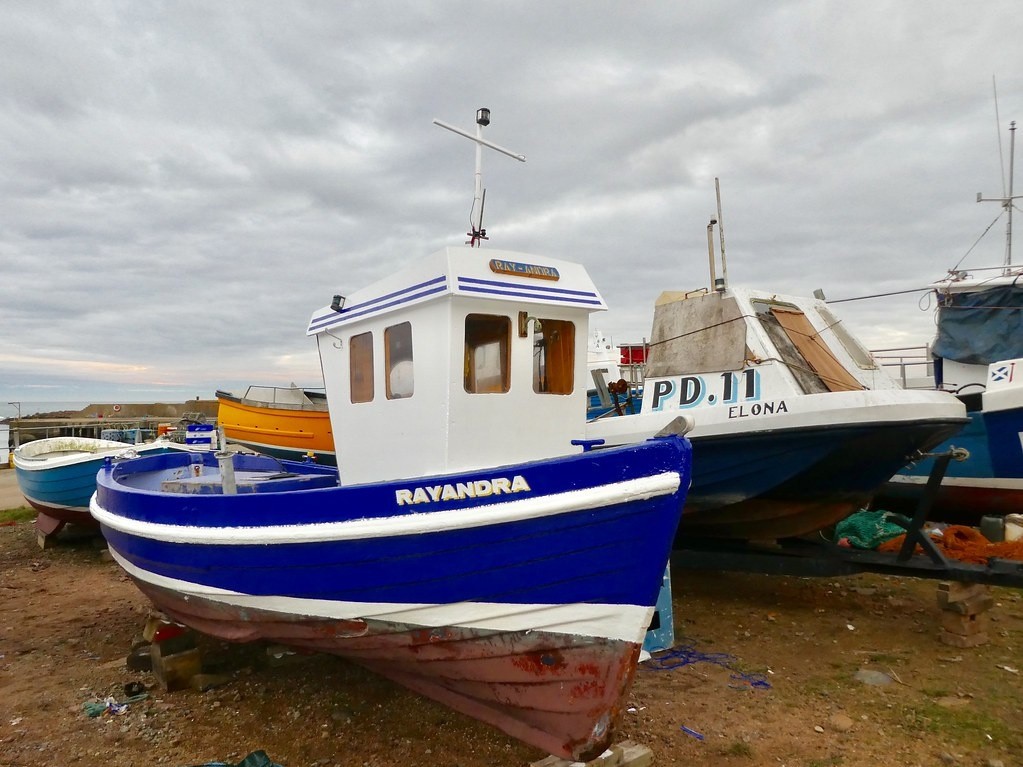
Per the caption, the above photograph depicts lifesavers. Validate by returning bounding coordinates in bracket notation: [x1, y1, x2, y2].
[113, 404, 121, 412]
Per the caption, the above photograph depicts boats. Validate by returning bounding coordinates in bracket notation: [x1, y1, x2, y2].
[12, 436, 201, 536]
[213, 361, 544, 467]
[91, 108, 693, 767]
[578, 171, 1023, 555]
